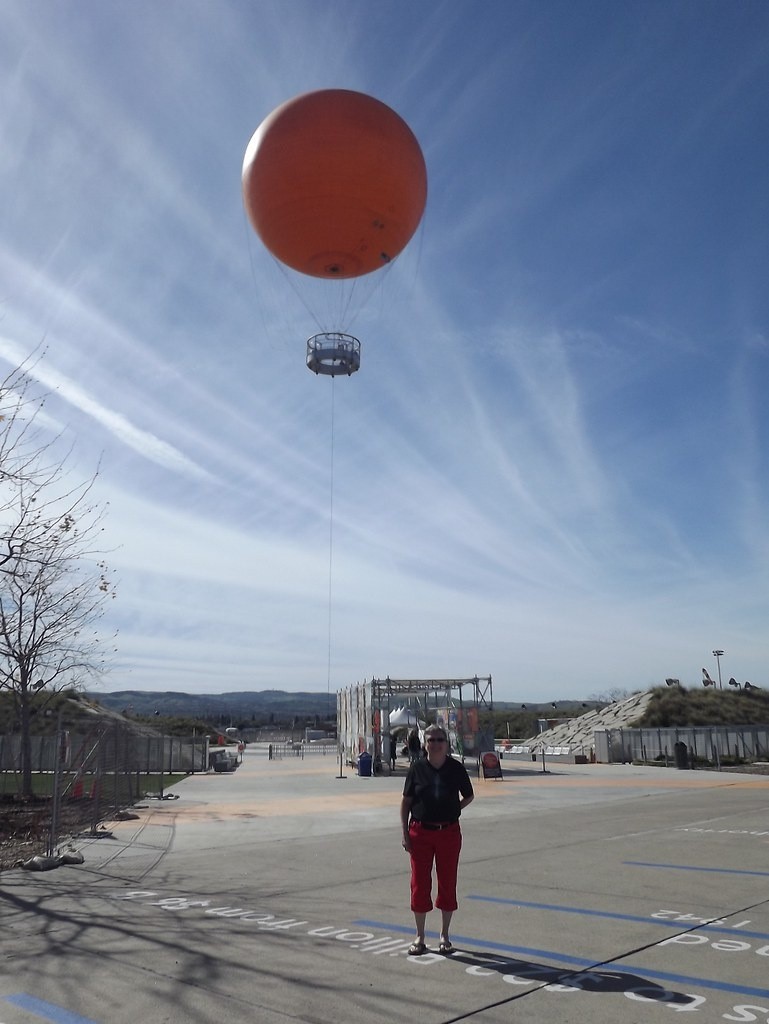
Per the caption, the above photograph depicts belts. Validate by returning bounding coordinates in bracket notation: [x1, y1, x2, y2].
[412, 816, 459, 831]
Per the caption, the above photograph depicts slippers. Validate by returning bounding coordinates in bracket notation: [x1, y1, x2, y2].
[408, 943, 427, 956]
[438, 940, 453, 954]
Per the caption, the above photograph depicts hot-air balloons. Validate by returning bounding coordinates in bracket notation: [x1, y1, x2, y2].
[243, 85, 430, 381]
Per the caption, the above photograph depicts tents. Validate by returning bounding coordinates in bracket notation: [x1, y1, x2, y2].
[387, 704, 427, 730]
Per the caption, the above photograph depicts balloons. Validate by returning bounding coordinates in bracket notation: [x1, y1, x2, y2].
[241, 88, 428, 280]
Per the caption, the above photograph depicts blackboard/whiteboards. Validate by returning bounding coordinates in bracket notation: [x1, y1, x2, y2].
[479, 752, 502, 779]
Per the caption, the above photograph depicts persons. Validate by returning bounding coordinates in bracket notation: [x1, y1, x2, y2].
[399, 723, 474, 956]
[409, 731, 421, 764]
[389, 737, 398, 771]
[407, 730, 416, 762]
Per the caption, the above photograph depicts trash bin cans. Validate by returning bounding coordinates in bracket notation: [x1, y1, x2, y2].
[357, 752, 373, 776]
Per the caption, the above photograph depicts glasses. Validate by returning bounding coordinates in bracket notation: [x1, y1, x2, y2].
[426, 737, 447, 743]
